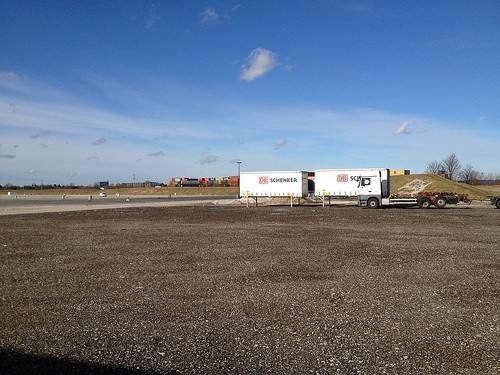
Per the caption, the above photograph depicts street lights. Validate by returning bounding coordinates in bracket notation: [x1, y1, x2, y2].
[237, 162, 242, 198]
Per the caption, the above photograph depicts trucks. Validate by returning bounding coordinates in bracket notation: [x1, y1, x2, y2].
[100, 192, 107, 197]
